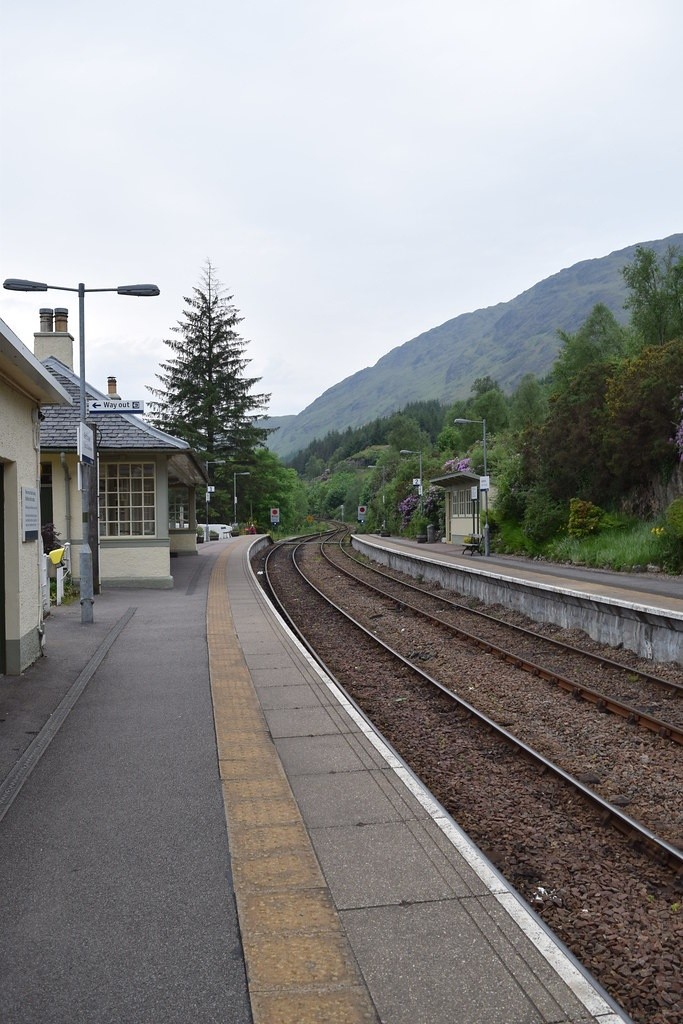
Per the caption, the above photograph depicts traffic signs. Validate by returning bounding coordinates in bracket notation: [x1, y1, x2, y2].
[88, 400, 144, 413]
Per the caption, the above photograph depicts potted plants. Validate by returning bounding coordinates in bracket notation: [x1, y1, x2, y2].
[231, 529, 239, 537]
[210, 529, 219, 540]
[380, 529, 391, 537]
[412, 511, 432, 543]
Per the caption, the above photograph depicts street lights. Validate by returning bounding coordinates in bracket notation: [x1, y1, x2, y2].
[453, 416, 490, 557]
[233, 471, 251, 534]
[399, 449, 423, 497]
[3, 277, 161, 625]
[368, 465, 389, 536]
[205, 460, 226, 543]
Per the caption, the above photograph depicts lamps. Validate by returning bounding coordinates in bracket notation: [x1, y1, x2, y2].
[49, 548, 65, 564]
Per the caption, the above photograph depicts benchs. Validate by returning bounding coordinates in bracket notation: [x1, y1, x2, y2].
[461, 534, 483, 556]
